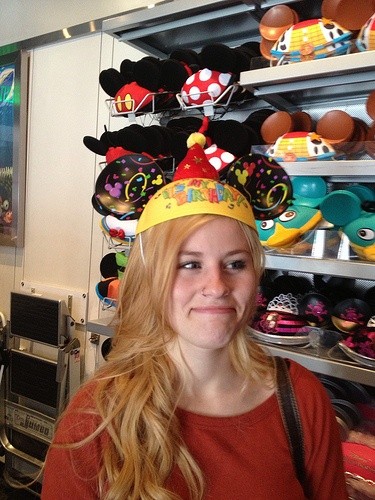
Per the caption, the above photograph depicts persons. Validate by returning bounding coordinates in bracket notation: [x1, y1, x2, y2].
[4, 130, 348, 500]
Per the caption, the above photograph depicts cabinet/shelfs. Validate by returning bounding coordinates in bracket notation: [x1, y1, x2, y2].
[87, 0, 375, 387]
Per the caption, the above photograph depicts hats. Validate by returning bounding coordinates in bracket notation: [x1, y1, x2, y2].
[99, 0, 375, 162]
[245, 275, 375, 369]
[253, 176, 375, 262]
[91, 132, 293, 236]
[96, 250, 129, 306]
